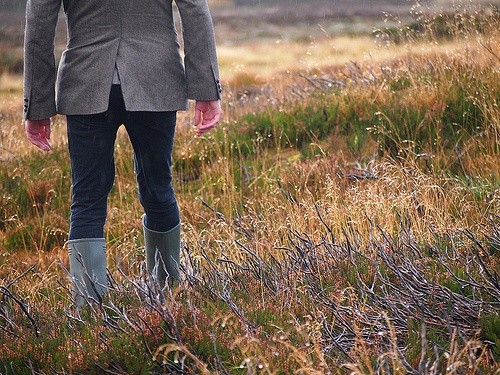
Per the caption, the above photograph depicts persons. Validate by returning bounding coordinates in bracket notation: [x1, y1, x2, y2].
[21, 0, 224, 309]
